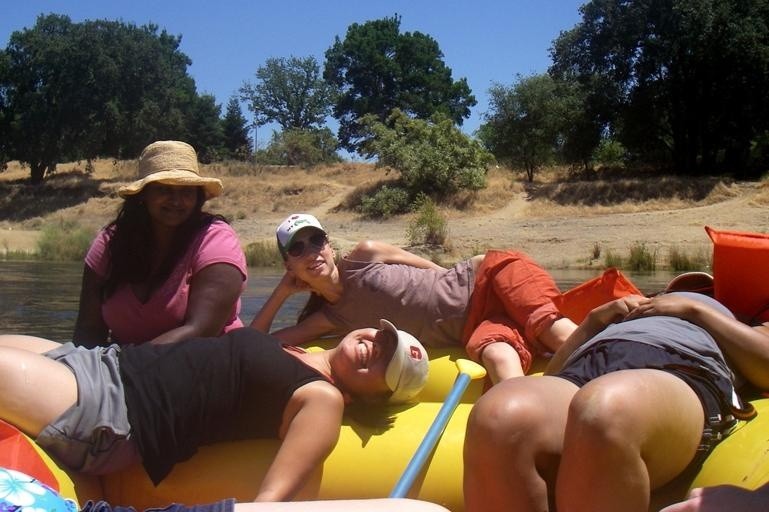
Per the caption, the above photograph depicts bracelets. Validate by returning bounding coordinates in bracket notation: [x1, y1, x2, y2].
[105, 327, 114, 344]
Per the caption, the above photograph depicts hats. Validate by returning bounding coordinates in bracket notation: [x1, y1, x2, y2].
[119, 141, 224, 201]
[663, 271, 714, 293]
[276, 213, 326, 258]
[380, 318, 429, 402]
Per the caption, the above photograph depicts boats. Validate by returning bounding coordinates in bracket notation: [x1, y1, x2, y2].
[0, 321, 769, 512]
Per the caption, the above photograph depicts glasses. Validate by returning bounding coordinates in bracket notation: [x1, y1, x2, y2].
[286, 235, 325, 257]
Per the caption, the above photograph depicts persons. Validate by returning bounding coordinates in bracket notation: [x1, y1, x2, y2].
[661, 480, 769, 511]
[462, 270, 769, 512]
[0, 316, 429, 501]
[249, 212, 580, 387]
[0, 467, 450, 512]
[71, 139, 248, 348]
